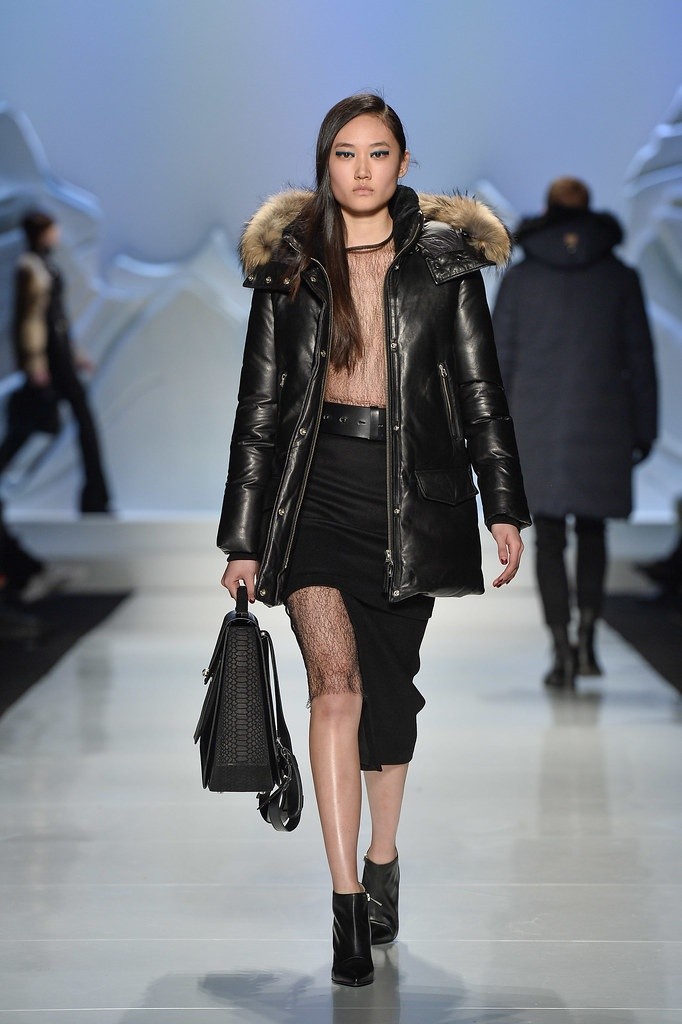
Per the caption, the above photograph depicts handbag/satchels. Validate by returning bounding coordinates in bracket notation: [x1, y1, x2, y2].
[192, 586, 303, 831]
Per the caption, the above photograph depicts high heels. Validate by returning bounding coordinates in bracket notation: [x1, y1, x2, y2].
[362, 846, 400, 945]
[332, 881, 376, 987]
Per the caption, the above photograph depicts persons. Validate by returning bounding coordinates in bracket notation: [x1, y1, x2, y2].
[216, 92, 534, 989]
[492, 178, 663, 692]
[0, 207, 113, 516]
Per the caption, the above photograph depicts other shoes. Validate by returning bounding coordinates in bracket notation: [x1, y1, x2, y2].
[542, 645, 576, 688]
[573, 640, 598, 675]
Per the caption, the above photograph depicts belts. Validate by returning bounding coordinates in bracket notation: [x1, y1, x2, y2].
[321, 402, 385, 440]
